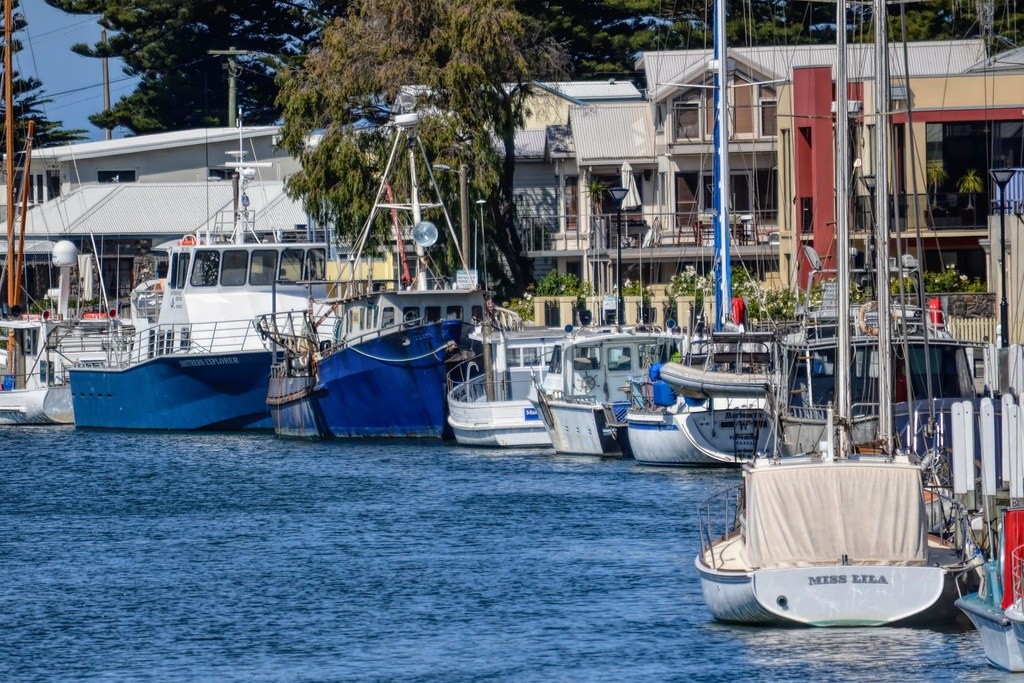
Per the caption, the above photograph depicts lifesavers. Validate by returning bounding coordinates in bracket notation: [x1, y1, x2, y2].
[83, 312, 106, 319]
[177, 239, 205, 246]
[859, 297, 883, 336]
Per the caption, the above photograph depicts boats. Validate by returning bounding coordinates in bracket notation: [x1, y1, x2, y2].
[446, 321, 683, 457]
[954, 506, 1024, 673]
[68, 105, 474, 438]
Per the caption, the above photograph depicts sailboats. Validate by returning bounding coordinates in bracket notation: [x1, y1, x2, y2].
[0, 0, 74, 426]
[623, 0, 979, 467]
[696, 0, 967, 629]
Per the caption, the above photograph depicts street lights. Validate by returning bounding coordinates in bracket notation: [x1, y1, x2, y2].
[989, 168, 1017, 348]
[608, 188, 629, 322]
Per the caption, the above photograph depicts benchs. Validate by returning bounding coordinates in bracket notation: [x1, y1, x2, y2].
[692, 214, 745, 246]
[612, 218, 652, 245]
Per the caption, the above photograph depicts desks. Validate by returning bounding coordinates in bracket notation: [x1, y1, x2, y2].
[704, 214, 752, 247]
